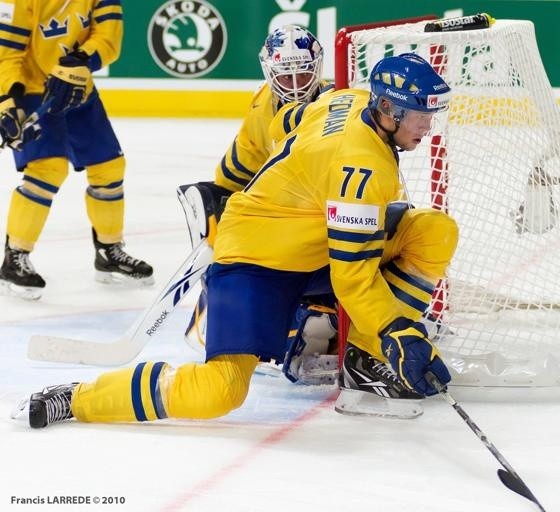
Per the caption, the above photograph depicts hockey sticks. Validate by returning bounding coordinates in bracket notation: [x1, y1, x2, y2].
[27, 239, 215, 368]
[421, 371, 546, 512]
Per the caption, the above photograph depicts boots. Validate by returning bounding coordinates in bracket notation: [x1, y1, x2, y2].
[92, 226, 153, 277]
[0, 234, 46, 287]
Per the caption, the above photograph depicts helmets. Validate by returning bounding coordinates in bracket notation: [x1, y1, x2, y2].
[368, 53, 452, 137]
[259, 24, 324, 104]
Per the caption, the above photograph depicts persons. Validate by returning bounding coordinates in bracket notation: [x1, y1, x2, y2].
[28, 53, 462, 427]
[177, 24, 345, 356]
[0, 0, 155, 288]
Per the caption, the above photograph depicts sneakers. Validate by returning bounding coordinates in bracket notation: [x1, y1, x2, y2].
[339, 341, 425, 399]
[29, 383, 80, 428]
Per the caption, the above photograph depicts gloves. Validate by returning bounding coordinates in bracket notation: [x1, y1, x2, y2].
[42, 51, 94, 115]
[378, 316, 451, 396]
[0, 94, 42, 153]
